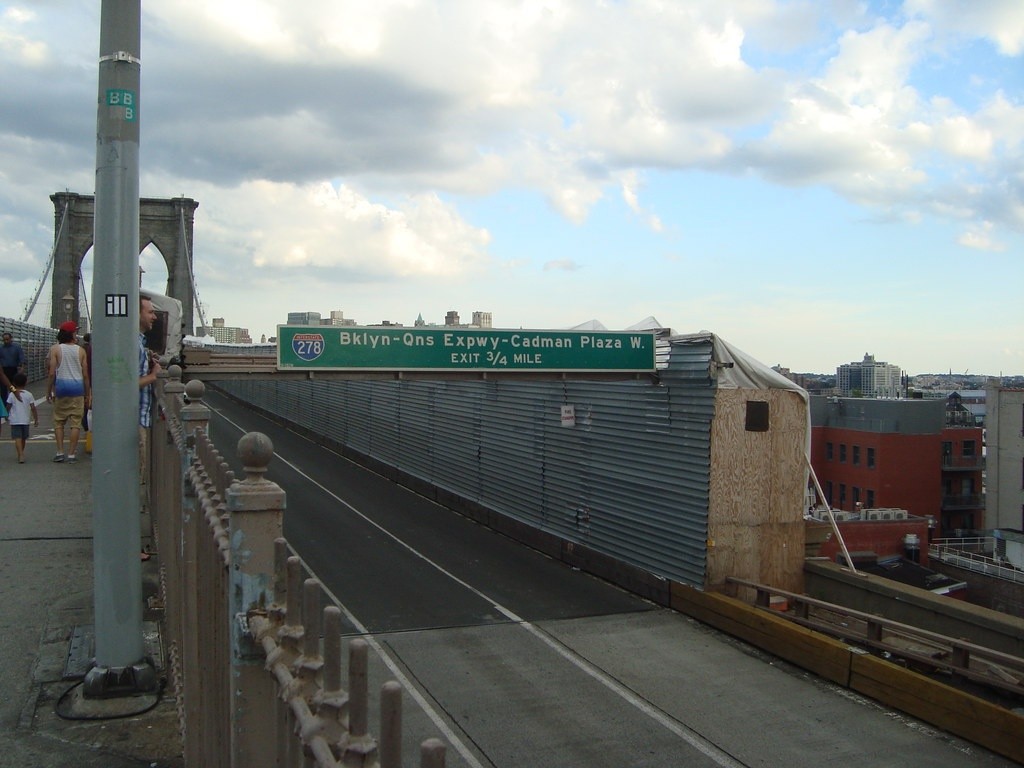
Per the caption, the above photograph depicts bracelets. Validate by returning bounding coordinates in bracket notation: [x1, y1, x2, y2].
[9, 385, 16, 392]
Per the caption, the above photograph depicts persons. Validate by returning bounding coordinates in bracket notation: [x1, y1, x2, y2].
[45, 321, 92, 462]
[139, 292, 162, 562]
[0, 333, 39, 463]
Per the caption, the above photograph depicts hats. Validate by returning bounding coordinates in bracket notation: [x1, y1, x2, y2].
[60, 321, 78, 331]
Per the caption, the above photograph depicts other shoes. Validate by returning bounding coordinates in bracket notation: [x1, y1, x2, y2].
[51, 453, 64, 462]
[68, 457, 80, 463]
